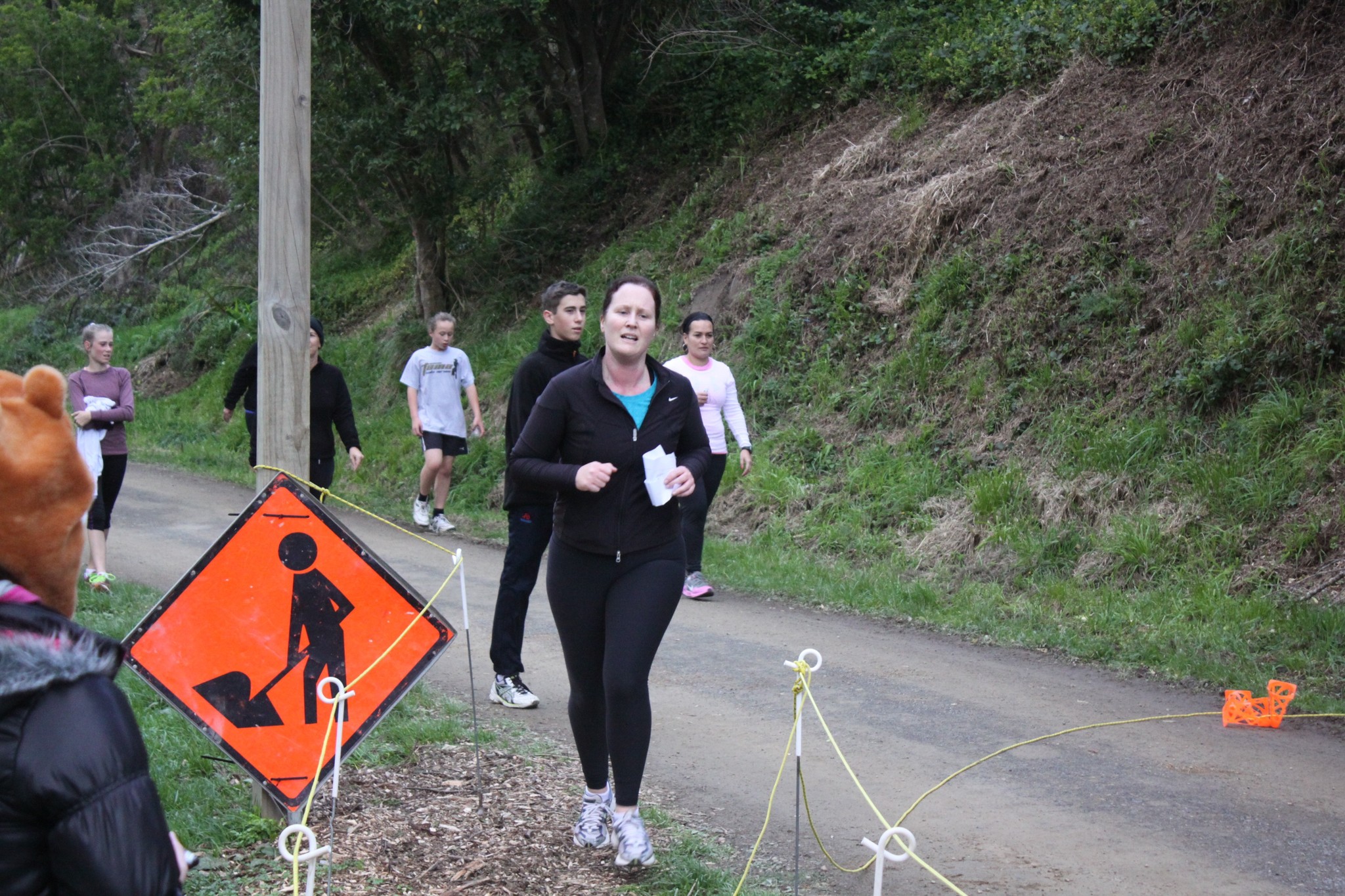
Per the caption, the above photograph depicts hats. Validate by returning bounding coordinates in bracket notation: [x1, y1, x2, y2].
[310, 317, 324, 347]
[0, 364, 95, 621]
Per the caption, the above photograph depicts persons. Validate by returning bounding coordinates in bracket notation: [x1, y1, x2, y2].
[309, 318, 363, 503]
[662, 312, 753, 598]
[67, 323, 135, 592]
[509, 275, 712, 867]
[490, 280, 590, 708]
[222, 341, 257, 469]
[399, 311, 484, 533]
[0, 363, 190, 896]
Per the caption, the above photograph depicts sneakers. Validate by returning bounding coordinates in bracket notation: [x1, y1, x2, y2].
[431, 513, 455, 532]
[682, 571, 714, 599]
[609, 810, 656, 867]
[84, 571, 96, 582]
[90, 571, 115, 595]
[489, 673, 539, 708]
[573, 780, 617, 849]
[414, 494, 430, 525]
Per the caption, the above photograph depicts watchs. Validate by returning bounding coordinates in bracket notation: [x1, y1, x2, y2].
[742, 447, 753, 454]
